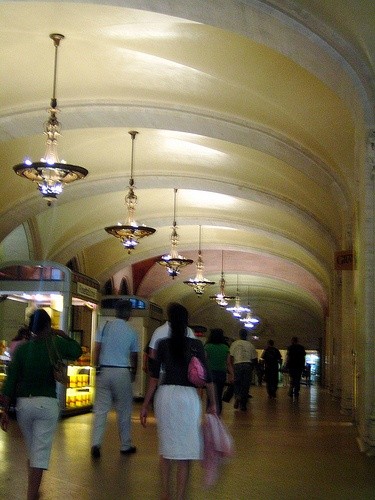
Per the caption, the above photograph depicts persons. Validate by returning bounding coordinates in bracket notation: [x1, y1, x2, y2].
[139, 303, 217, 500]
[91, 300, 138, 456]
[142, 303, 312, 419]
[0, 309, 82, 500]
[10, 327, 32, 361]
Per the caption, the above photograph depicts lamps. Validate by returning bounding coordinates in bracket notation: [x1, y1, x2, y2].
[13, 34, 88, 206]
[182, 224, 216, 297]
[226, 275, 251, 320]
[151, 188, 193, 279]
[239, 288, 261, 328]
[104, 130, 156, 255]
[210, 250, 236, 310]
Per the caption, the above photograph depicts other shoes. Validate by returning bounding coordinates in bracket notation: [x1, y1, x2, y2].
[122, 446, 136, 454]
[241, 406, 247, 411]
[234, 398, 241, 409]
[90, 446, 102, 459]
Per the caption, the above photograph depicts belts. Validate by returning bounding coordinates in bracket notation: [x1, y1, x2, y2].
[101, 365, 128, 369]
[20, 394, 58, 400]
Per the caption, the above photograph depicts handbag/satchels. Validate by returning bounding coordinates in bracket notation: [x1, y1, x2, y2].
[45, 335, 67, 386]
[189, 357, 206, 388]
